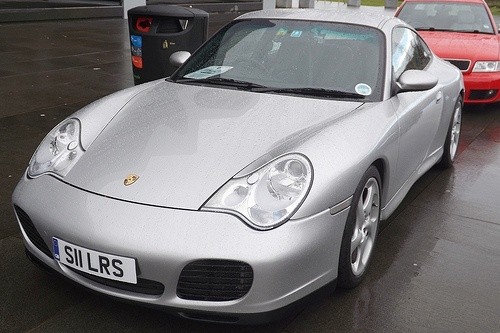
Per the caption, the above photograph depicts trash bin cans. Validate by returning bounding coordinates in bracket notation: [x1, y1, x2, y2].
[127, 5, 210, 86]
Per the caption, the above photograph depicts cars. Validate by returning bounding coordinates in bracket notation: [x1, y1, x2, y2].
[11, 5, 465, 315]
[393, 0, 500, 106]
